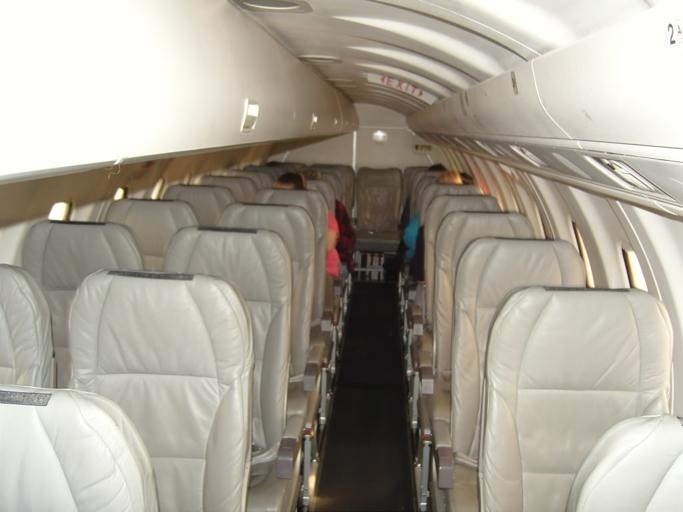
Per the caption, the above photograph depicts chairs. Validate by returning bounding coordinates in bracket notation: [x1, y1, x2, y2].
[0, 161, 355, 512]
[399, 167, 683, 512]
[356, 168, 403, 253]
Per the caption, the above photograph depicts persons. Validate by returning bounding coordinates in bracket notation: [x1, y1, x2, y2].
[298, 168, 357, 269]
[380, 160, 478, 290]
[270, 171, 341, 277]
